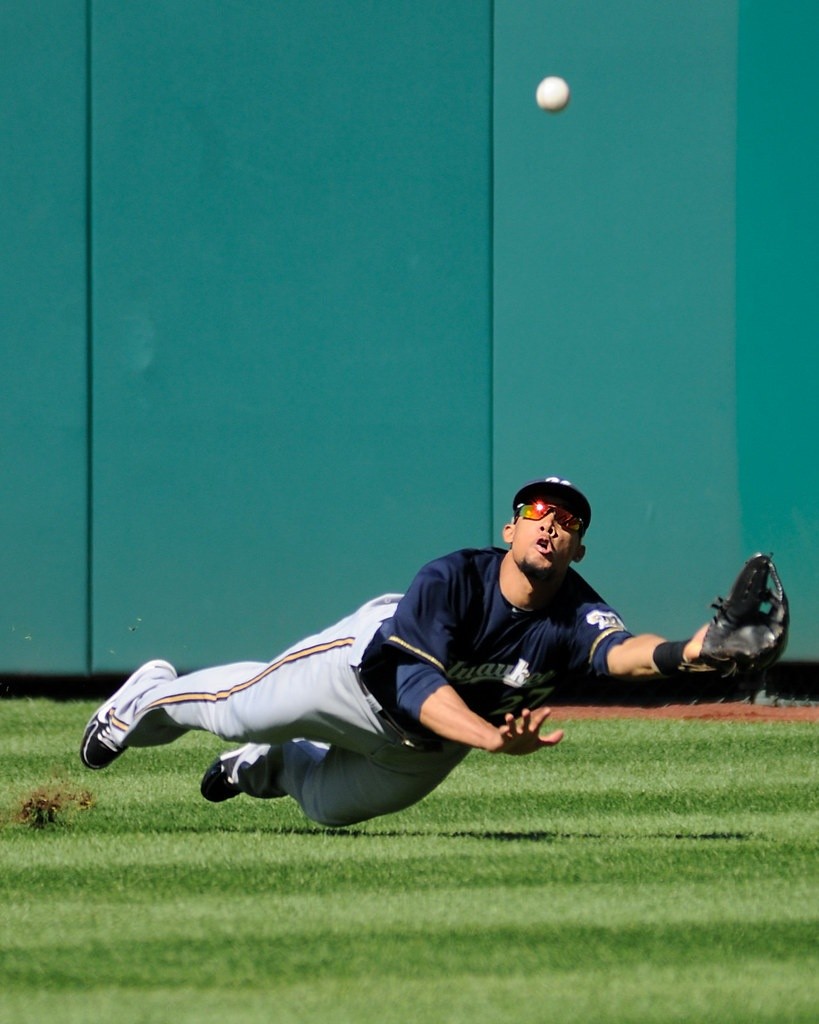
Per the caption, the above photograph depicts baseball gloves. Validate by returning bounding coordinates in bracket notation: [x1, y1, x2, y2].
[698, 554, 790, 678]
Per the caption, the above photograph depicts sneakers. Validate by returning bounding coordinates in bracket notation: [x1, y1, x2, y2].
[201, 743, 258, 803]
[80, 659, 179, 770]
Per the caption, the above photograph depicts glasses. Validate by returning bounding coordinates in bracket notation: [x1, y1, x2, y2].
[513, 502, 585, 536]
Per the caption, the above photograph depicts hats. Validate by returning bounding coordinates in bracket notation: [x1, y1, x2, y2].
[512, 476, 592, 538]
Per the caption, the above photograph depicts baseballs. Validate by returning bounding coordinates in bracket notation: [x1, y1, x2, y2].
[536, 76, 569, 112]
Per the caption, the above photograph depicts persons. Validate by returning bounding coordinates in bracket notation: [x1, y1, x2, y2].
[77, 475, 790, 829]
